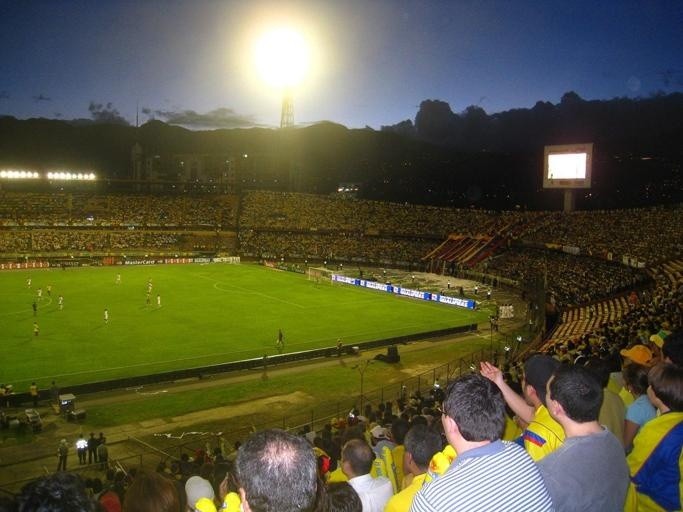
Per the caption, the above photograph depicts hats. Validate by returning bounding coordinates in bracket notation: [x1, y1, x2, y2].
[185, 475, 215, 511]
[573, 355, 610, 387]
[524, 355, 561, 408]
[620, 345, 653, 367]
[369, 426, 391, 440]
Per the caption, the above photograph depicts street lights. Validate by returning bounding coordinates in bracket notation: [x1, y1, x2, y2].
[355, 360, 370, 419]
[486, 312, 499, 367]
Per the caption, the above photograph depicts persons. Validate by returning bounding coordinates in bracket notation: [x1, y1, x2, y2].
[114, 272, 121, 286]
[57, 439, 71, 472]
[49, 380, 59, 402]
[1, 448, 242, 510]
[30, 301, 38, 317]
[1, 187, 242, 260]
[25, 277, 31, 288]
[238, 190, 679, 277]
[231, 388, 683, 510]
[76, 433, 109, 470]
[275, 326, 285, 348]
[36, 287, 43, 302]
[0, 383, 9, 407]
[142, 276, 154, 311]
[411, 273, 415, 282]
[46, 284, 51, 297]
[30, 380, 39, 407]
[446, 279, 451, 289]
[469, 274, 683, 391]
[103, 309, 109, 325]
[56, 295, 64, 312]
[154, 294, 162, 308]
[32, 321, 40, 336]
